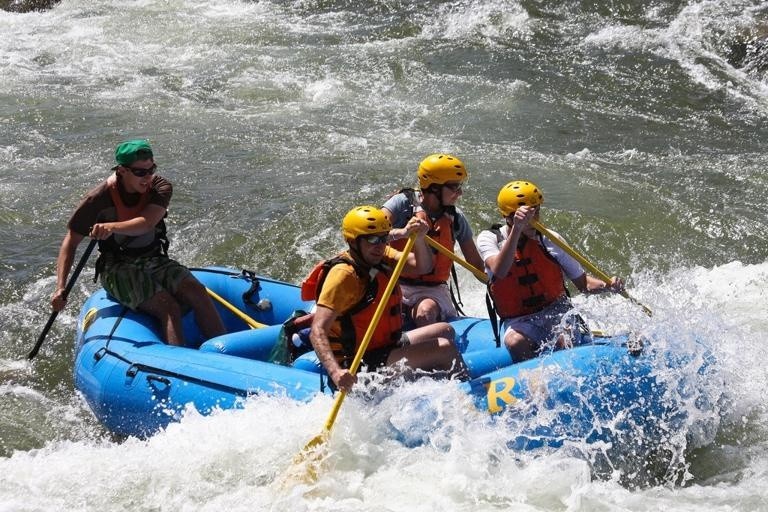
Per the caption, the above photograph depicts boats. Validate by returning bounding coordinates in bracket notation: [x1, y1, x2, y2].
[69, 265, 717, 473]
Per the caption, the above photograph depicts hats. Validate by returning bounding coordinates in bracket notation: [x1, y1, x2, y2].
[110, 140, 153, 171]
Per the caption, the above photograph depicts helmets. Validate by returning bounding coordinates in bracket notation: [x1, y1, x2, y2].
[342, 205, 392, 242]
[417, 154, 466, 189]
[497, 180, 544, 217]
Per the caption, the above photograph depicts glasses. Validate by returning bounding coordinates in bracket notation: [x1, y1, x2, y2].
[122, 163, 156, 176]
[445, 183, 463, 191]
[361, 234, 392, 244]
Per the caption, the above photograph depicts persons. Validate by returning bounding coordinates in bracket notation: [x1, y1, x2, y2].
[309, 206, 457, 394]
[476, 180, 625, 365]
[49, 139, 225, 347]
[380, 154, 484, 327]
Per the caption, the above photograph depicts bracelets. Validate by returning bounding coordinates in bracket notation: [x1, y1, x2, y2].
[392, 228, 398, 241]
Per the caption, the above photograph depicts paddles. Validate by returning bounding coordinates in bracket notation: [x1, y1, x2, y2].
[278, 217, 418, 490]
